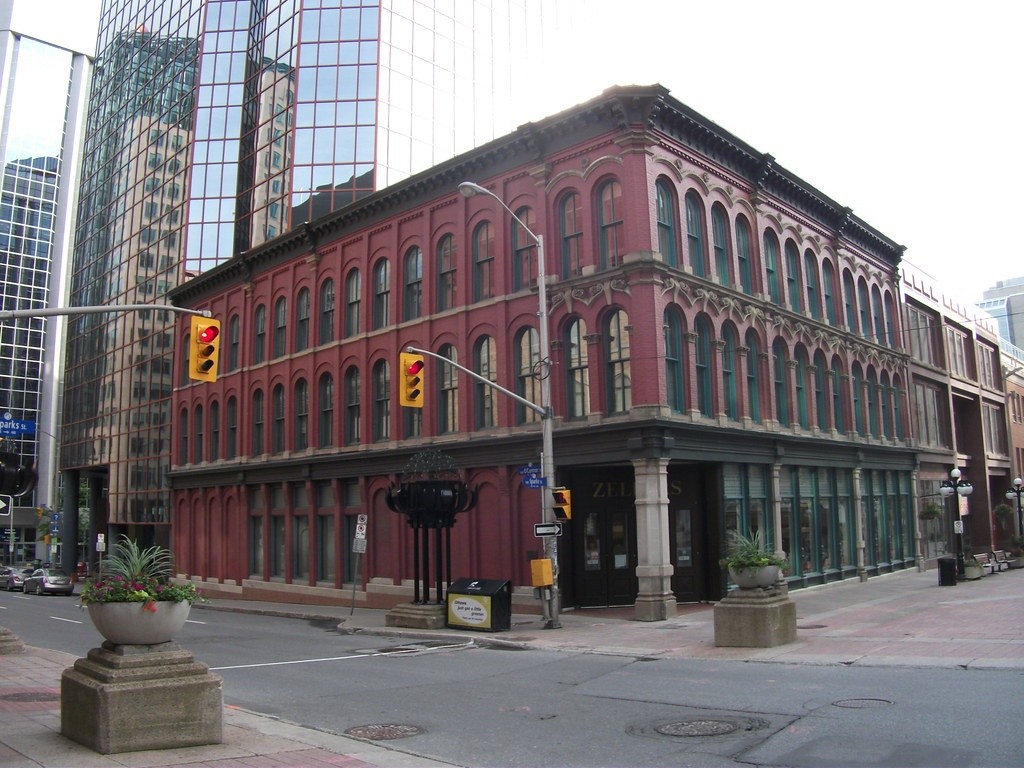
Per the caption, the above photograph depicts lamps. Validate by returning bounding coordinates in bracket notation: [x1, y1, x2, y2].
[1005, 366, 1023, 378]
[1009, 390, 1016, 399]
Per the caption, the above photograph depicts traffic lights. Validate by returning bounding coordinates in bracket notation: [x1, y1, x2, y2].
[551, 490, 572, 520]
[189, 314, 221, 382]
[399, 352, 424, 408]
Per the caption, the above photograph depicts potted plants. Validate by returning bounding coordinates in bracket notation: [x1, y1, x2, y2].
[964, 561, 986, 579]
[383, 447, 490, 517]
[718, 527, 792, 588]
[920, 503, 944, 520]
[1007, 547, 1024, 567]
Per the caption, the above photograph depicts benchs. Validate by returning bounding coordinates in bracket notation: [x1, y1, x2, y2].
[972, 552, 1001, 574]
[991, 550, 1016, 572]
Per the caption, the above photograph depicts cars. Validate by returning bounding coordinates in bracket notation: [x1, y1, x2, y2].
[0, 567, 33, 592]
[22, 567, 74, 596]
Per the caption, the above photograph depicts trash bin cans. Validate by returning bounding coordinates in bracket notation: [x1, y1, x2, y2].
[445, 578, 512, 633]
[937, 558, 958, 586]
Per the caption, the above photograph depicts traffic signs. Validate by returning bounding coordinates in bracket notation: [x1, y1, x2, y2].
[533, 522, 564, 538]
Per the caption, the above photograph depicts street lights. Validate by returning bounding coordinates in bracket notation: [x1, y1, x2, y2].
[456, 180, 564, 630]
[1005, 477, 1024, 542]
[940, 468, 973, 582]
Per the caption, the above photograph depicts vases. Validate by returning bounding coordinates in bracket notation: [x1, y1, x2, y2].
[88, 598, 192, 644]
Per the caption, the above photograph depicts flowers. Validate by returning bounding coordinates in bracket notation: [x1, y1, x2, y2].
[73, 535, 211, 613]
